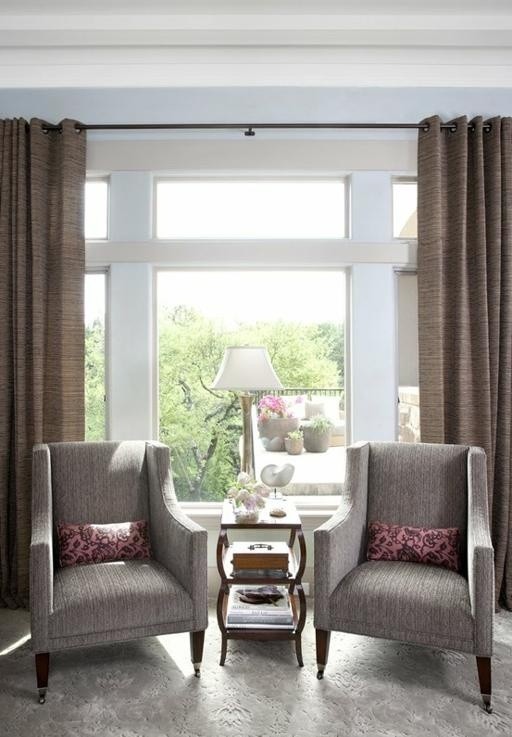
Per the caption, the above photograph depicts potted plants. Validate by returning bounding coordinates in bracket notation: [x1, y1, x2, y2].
[300, 414, 333, 452]
[284, 430, 304, 454]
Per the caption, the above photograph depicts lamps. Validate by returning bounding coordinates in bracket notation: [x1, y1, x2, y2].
[210, 344, 283, 480]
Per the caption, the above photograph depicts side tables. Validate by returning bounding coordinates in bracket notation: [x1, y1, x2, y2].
[217, 499, 307, 667]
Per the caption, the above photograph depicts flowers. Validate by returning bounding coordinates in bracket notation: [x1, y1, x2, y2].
[229, 472, 271, 510]
[256, 395, 303, 421]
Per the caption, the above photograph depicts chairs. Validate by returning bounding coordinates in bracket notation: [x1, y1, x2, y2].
[30, 441, 209, 704]
[313, 441, 495, 713]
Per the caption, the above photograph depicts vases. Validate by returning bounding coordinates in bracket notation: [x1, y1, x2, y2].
[258, 419, 299, 451]
[235, 504, 259, 524]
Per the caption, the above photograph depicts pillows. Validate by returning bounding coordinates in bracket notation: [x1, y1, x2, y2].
[366, 521, 459, 572]
[56, 520, 155, 568]
